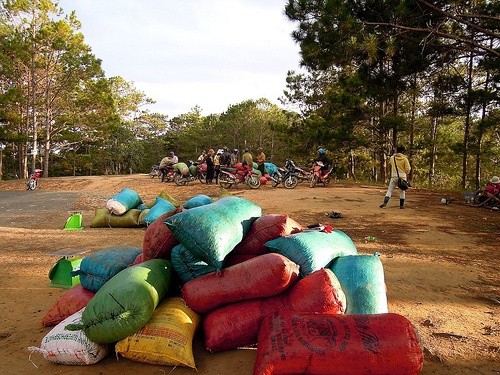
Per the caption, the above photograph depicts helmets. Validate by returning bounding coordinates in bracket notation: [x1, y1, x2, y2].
[318, 149, 325, 155]
[233, 149, 239, 154]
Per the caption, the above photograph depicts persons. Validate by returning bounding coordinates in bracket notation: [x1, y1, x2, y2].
[475, 176, 500, 209]
[307, 148, 328, 170]
[159, 151, 178, 183]
[379, 146, 411, 209]
[198, 145, 253, 184]
[256, 147, 265, 176]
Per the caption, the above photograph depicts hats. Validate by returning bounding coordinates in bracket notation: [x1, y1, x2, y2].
[490, 175, 500, 184]
[257, 147, 263, 151]
[245, 148, 248, 151]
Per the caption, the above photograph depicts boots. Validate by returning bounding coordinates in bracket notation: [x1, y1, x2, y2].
[400, 199, 405, 209]
[379, 196, 390, 208]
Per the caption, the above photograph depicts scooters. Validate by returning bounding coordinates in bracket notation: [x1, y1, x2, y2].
[308, 159, 335, 188]
[25, 168, 44, 191]
[148, 156, 313, 189]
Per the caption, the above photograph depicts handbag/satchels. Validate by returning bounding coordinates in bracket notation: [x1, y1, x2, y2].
[397, 178, 412, 190]
